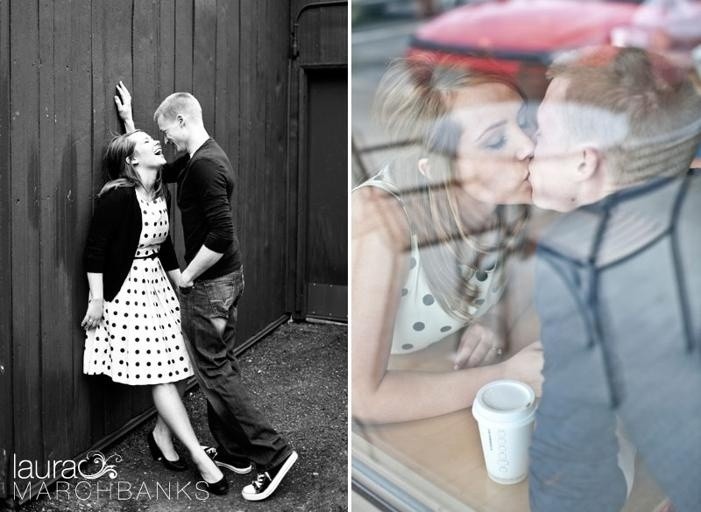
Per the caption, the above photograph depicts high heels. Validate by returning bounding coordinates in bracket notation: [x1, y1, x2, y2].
[196, 452, 228, 496]
[147, 427, 186, 472]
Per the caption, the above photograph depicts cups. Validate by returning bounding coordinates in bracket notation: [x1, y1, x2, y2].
[472, 381, 537, 486]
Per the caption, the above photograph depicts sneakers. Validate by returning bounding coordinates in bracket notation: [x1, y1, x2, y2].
[241, 450, 298, 501]
[200, 446, 252, 475]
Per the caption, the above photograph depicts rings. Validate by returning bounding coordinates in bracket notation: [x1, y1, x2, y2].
[88, 317, 95, 326]
[493, 344, 504, 358]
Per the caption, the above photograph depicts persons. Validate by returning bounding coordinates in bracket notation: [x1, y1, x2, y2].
[351, 45, 546, 429]
[113, 79, 299, 502]
[529, 49, 701, 511]
[78, 129, 230, 498]
